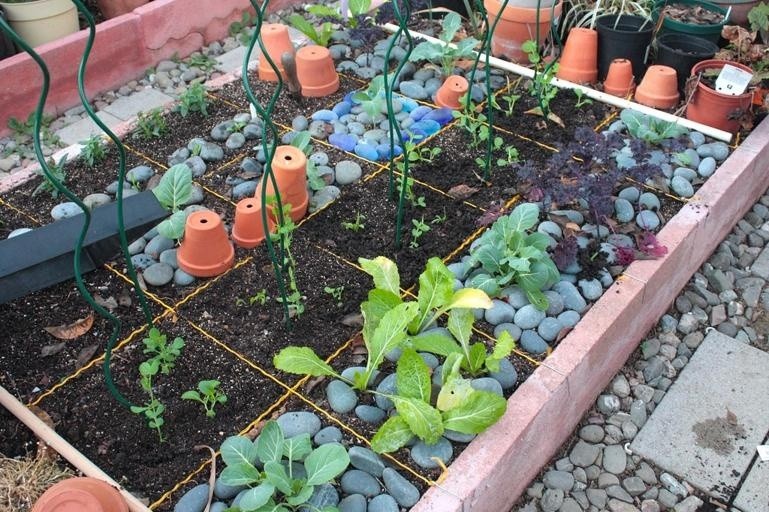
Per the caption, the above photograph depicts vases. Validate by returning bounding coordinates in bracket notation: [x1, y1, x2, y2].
[484, 0, 769, 139]
[96, 0, 154, 20]
[433, 74, 469, 110]
[258, 24, 296, 81]
[233, 198, 277, 249]
[31, 478, 129, 512]
[295, 45, 340, 98]
[176, 210, 235, 277]
[255, 146, 309, 224]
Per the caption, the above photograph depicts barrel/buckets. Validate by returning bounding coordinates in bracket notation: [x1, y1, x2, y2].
[656, 33, 720, 90]
[685, 59, 757, 134]
[651, 0, 730, 46]
[597, 14, 654, 76]
